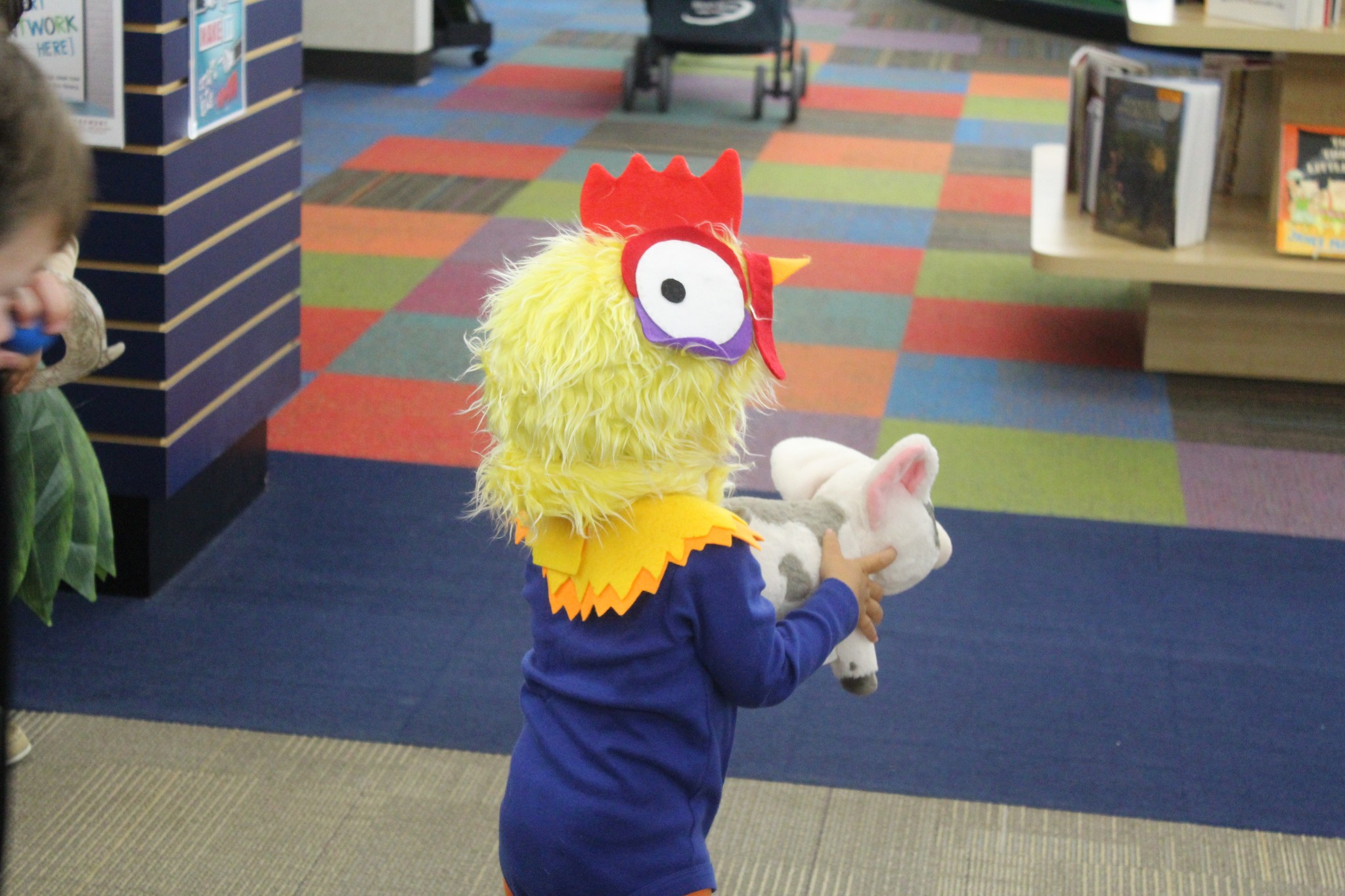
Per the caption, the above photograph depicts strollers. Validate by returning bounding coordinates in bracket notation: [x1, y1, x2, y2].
[621, 0, 809, 123]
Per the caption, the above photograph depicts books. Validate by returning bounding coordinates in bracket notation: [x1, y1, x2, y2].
[1065, 0, 1345, 258]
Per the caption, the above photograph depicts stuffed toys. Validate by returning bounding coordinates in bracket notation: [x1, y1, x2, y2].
[469, 143, 954, 896]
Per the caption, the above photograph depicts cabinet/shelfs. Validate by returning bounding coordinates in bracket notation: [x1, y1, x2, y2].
[1030, 0, 1345, 384]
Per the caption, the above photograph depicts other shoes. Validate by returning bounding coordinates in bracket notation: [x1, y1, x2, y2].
[6, 725, 32, 766]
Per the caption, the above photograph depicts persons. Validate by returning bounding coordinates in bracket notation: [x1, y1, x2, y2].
[0, 0, 92, 887]
